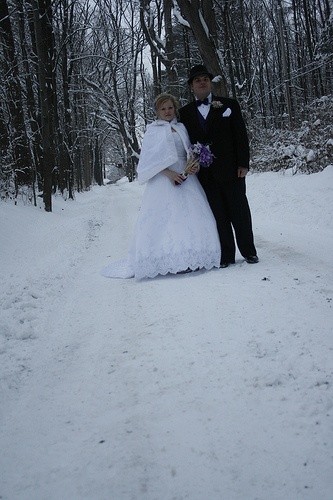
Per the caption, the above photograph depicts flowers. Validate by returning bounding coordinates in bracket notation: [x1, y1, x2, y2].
[174, 142, 216, 185]
[211, 100, 224, 109]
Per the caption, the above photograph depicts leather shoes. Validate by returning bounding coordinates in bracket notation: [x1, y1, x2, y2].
[247, 255, 259, 263]
[219, 259, 235, 268]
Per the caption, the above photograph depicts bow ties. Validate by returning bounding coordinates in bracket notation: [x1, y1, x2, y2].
[197, 98, 210, 106]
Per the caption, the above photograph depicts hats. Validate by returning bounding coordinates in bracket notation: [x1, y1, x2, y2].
[187, 64, 214, 85]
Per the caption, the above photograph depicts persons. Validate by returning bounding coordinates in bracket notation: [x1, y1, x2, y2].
[102, 92, 222, 277]
[178, 64, 260, 267]
